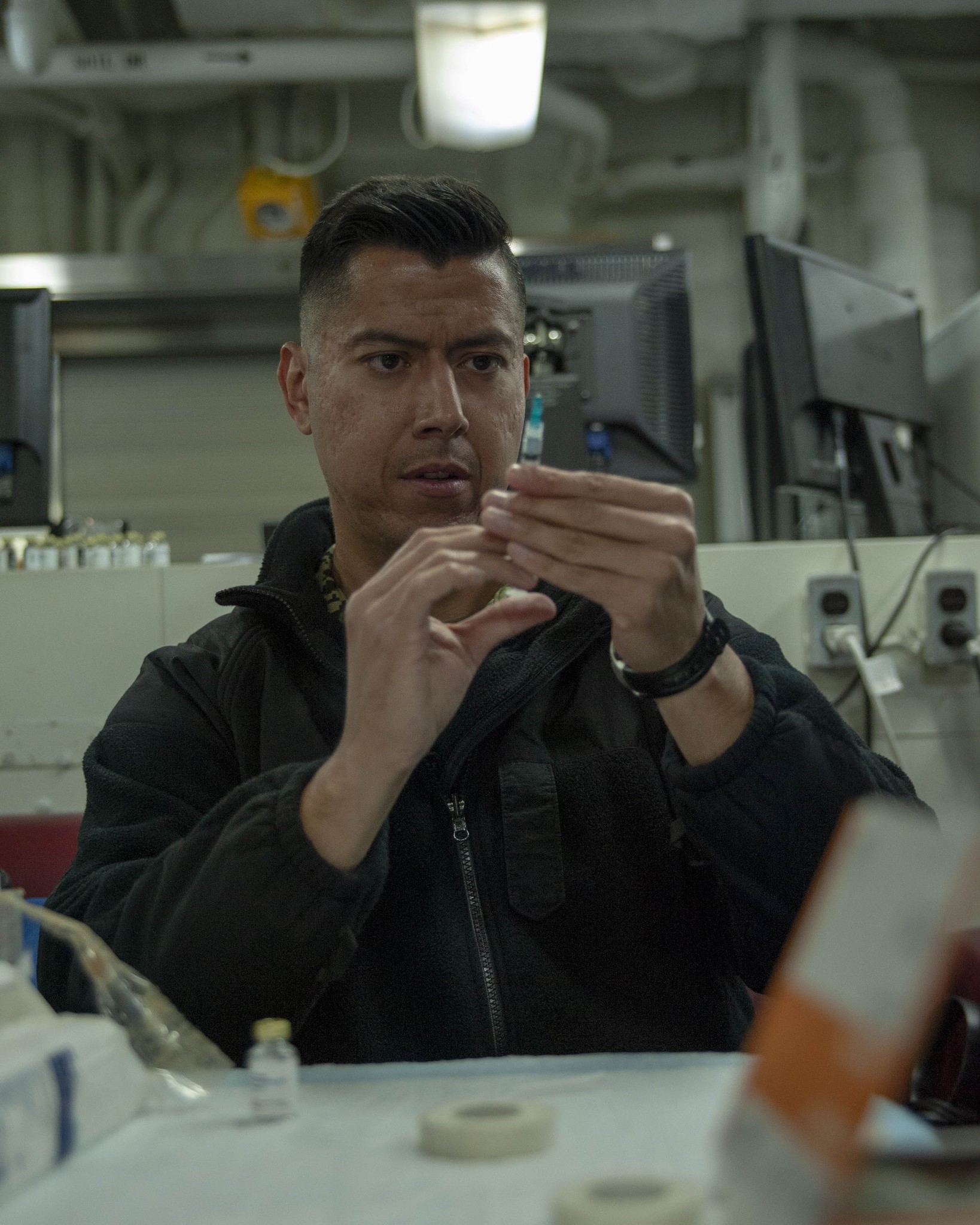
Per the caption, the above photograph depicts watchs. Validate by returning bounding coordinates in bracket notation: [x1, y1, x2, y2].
[607, 603, 733, 706]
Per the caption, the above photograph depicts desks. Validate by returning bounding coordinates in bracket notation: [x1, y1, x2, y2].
[1, 1052, 942, 1225]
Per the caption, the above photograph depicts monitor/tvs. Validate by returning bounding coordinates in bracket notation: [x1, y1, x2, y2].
[515, 246, 701, 484]
[0, 289, 53, 527]
[741, 230, 935, 543]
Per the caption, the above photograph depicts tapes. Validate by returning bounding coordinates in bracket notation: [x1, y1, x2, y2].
[557, 1171, 700, 1225]
[425, 1096, 551, 1160]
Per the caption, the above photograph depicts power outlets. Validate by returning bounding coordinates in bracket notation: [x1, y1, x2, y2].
[806, 573, 867, 672]
[922, 563, 979, 669]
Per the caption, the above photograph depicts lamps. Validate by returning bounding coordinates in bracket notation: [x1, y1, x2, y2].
[407, 0, 549, 157]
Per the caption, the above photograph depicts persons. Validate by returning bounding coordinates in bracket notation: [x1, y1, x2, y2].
[38, 173, 940, 1068]
